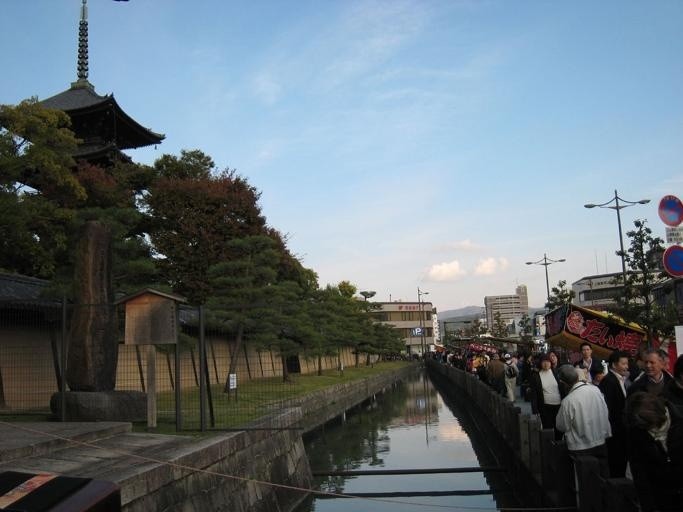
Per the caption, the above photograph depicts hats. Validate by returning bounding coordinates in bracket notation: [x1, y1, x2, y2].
[492, 353, 500, 360]
[556, 364, 579, 384]
[504, 353, 511, 358]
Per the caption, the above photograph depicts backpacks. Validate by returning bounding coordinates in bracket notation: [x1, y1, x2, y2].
[505, 362, 517, 379]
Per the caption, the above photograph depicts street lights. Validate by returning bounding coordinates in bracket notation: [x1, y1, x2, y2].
[582, 188, 651, 306]
[524, 252, 567, 314]
[416, 286, 429, 359]
